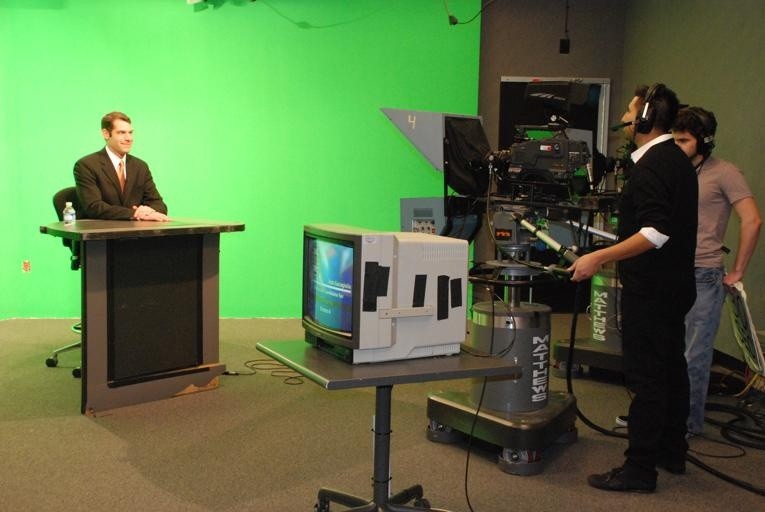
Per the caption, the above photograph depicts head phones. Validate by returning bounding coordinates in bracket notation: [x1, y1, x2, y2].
[636, 81, 666, 134]
[679, 108, 714, 155]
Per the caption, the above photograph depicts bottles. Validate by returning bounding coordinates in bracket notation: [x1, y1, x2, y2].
[64, 202, 77, 226]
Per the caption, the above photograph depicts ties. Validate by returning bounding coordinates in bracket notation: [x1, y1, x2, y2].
[117, 160, 126, 193]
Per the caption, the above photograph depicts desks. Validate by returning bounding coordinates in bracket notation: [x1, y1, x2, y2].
[254, 339, 522, 511]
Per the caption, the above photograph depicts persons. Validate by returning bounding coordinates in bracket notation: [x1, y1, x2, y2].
[615, 103, 762, 436]
[73, 111, 172, 222]
[567, 82, 698, 494]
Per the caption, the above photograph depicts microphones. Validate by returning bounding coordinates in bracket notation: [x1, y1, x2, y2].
[611, 121, 632, 131]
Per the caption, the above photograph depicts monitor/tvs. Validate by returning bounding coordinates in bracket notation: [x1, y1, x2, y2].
[302, 223, 469, 365]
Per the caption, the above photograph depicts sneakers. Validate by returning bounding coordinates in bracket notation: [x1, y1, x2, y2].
[616, 413, 628, 424]
[587, 463, 659, 494]
[656, 456, 685, 475]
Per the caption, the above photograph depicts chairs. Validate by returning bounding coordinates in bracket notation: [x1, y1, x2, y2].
[45, 186, 83, 378]
